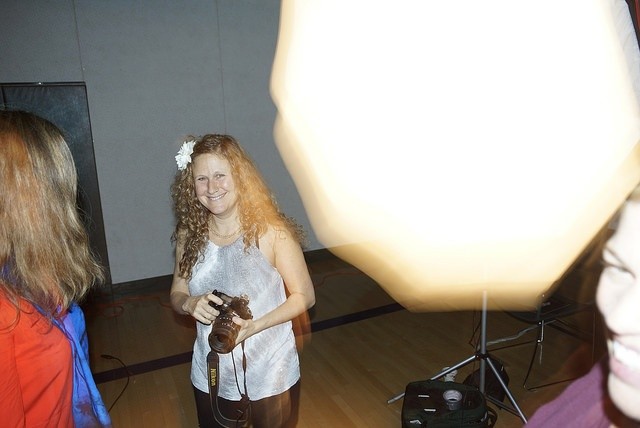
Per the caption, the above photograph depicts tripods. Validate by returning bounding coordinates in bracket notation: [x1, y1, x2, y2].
[387, 293, 527, 424]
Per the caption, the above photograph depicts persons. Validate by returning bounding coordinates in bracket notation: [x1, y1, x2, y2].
[522, 181, 639, 427]
[168, 133, 318, 427]
[1, 109, 113, 428]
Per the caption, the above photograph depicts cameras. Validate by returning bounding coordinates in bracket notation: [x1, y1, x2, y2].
[208, 289, 253, 354]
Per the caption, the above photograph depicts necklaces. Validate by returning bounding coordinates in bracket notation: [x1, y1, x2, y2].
[209, 223, 241, 239]
